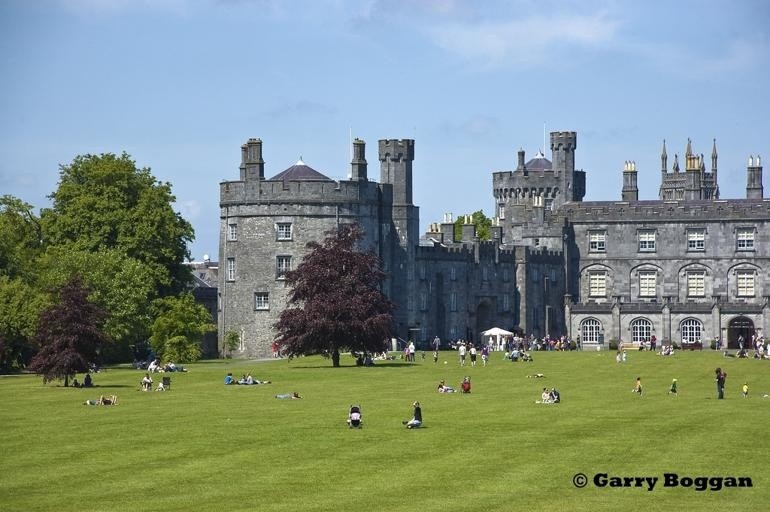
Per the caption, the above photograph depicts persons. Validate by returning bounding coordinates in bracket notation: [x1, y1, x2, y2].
[526, 373, 543, 378]
[632, 377, 642, 396]
[355, 332, 582, 368]
[71, 358, 188, 405]
[668, 379, 677, 396]
[275, 392, 302, 400]
[438, 380, 454, 392]
[223, 372, 270, 385]
[615, 335, 674, 364]
[542, 388, 554, 404]
[548, 388, 560, 403]
[714, 367, 727, 399]
[406, 400, 422, 429]
[271, 341, 284, 358]
[742, 382, 748, 399]
[715, 332, 770, 360]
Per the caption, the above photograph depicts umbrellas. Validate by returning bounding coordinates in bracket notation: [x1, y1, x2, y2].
[483, 327, 514, 347]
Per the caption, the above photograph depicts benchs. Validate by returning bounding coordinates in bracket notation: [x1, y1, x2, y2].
[622, 343, 639, 351]
[681, 343, 702, 351]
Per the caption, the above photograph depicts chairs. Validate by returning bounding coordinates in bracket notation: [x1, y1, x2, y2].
[163, 377, 170, 390]
